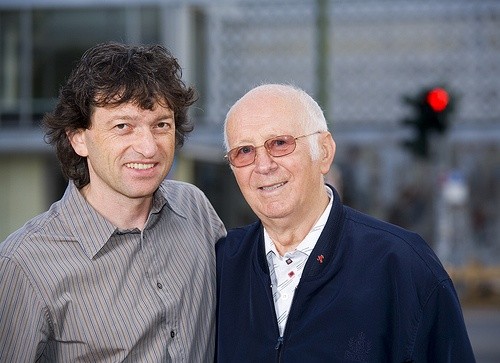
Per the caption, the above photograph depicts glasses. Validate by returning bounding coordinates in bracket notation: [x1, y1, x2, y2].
[224, 131, 321, 168]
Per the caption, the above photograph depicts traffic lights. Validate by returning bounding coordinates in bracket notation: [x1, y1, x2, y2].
[400, 85, 458, 160]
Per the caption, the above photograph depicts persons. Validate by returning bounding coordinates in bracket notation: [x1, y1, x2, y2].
[0, 40, 228, 362]
[214, 83, 477, 363]
